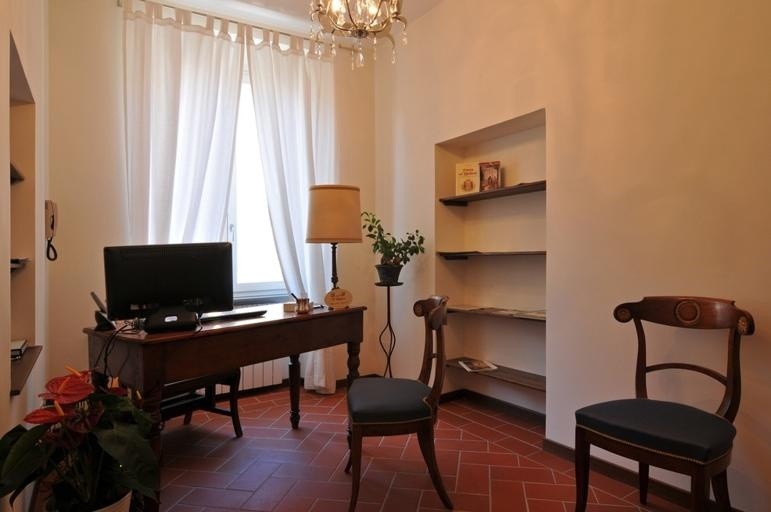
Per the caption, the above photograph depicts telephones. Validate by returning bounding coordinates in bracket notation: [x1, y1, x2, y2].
[91, 289, 115, 332]
[45, 199, 59, 262]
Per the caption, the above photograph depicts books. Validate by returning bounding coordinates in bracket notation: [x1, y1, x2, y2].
[455, 160, 501, 195]
[458, 359, 497, 373]
[11, 256, 28, 265]
[11, 338, 27, 362]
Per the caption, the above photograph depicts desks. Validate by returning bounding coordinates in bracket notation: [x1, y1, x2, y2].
[81, 300, 368, 440]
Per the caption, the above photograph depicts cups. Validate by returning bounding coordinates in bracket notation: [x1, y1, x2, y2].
[297, 298, 309, 314]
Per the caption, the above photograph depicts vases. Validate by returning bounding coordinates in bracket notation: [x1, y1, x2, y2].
[42, 487, 131, 511]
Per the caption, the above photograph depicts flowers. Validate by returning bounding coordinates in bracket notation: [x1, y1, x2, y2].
[0, 366, 164, 511]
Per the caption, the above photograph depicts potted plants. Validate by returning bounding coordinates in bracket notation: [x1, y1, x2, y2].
[360, 208, 426, 282]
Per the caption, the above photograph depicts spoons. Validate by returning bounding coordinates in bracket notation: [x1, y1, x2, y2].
[289, 293, 301, 301]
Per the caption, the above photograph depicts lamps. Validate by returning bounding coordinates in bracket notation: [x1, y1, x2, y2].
[304, 183, 365, 309]
[307, 0, 411, 70]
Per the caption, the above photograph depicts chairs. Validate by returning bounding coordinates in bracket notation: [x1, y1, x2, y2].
[342, 294, 457, 512]
[575, 294, 757, 512]
[158, 367, 243, 439]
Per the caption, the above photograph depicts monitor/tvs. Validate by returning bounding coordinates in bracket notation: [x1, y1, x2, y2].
[103, 243, 234, 331]
